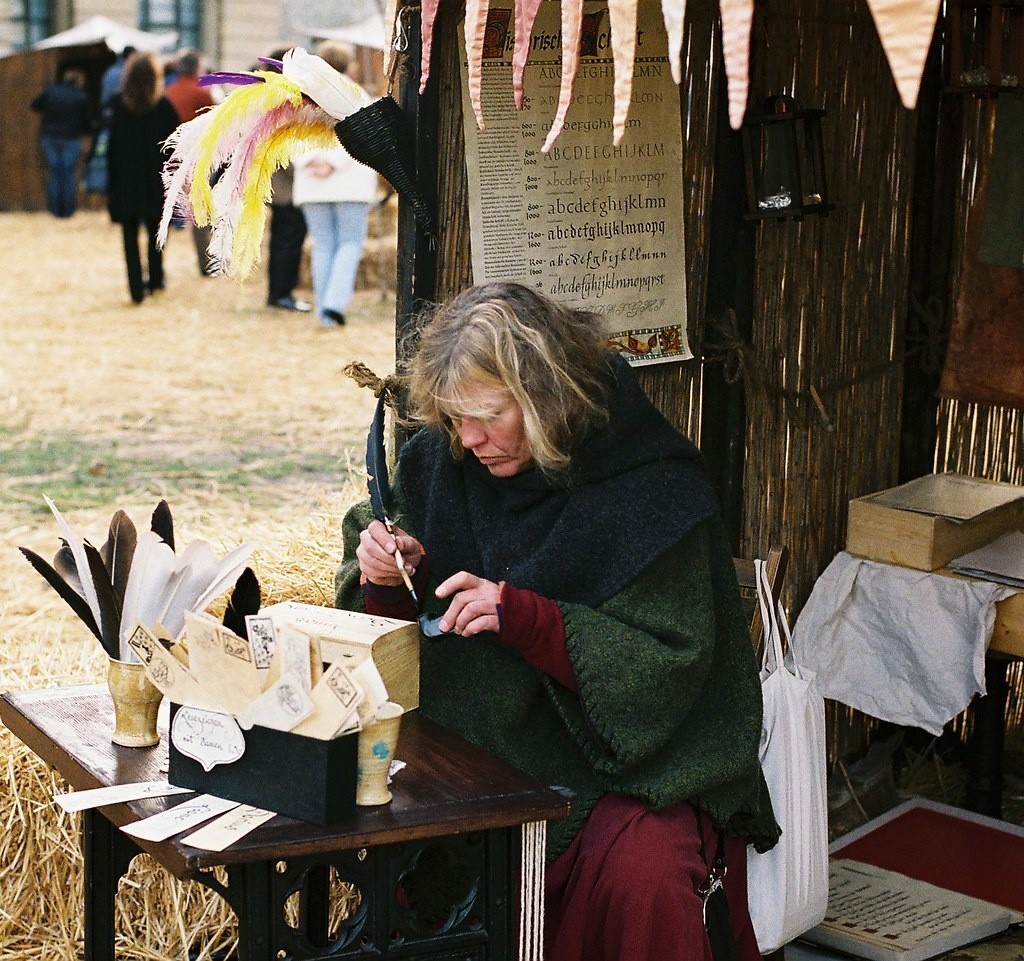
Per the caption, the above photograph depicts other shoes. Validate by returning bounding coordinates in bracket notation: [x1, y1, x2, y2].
[322, 308, 345, 327]
[148, 281, 165, 295]
[268, 295, 312, 312]
[132, 291, 143, 304]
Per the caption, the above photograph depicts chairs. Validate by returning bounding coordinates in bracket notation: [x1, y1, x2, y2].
[731, 545, 791, 662]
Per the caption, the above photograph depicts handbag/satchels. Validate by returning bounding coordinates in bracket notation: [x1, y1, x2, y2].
[747, 558, 830, 957]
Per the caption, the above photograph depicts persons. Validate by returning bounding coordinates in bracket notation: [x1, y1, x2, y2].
[101, 45, 311, 312]
[290, 41, 376, 325]
[336, 281, 782, 961]
[31, 70, 89, 219]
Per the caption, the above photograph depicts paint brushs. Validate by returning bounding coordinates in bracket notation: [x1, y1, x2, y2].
[14, 491, 265, 667]
[365, 385, 421, 604]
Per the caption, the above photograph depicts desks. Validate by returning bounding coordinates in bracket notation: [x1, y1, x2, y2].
[844, 553, 1024, 819]
[0, 684, 571, 961]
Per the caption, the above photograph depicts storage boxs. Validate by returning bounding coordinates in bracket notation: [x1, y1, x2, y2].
[257, 600, 422, 714]
[167, 702, 359, 829]
[846, 469, 1023, 572]
